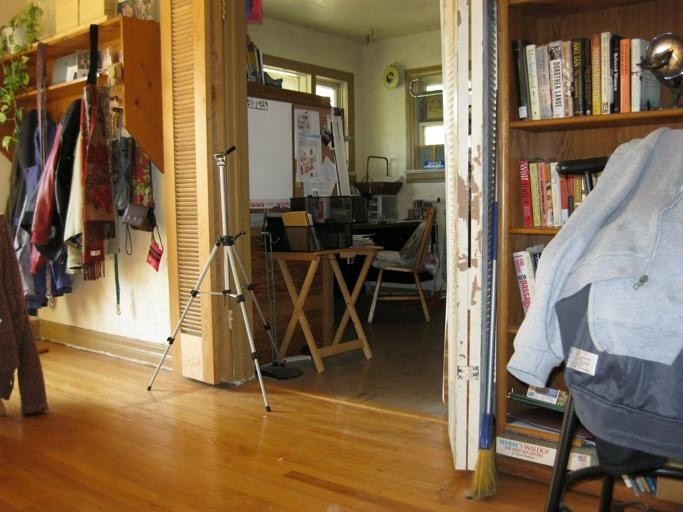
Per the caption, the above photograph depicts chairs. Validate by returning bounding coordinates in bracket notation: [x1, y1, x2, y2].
[367, 207, 434, 323]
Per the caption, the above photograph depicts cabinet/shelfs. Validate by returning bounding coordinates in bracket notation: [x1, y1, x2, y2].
[0, 16, 165, 174]
[495, 0, 682, 511]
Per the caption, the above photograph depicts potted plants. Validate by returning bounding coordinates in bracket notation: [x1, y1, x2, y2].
[0, 3, 44, 152]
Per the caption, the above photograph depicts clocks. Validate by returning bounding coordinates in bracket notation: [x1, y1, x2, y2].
[383, 64, 400, 89]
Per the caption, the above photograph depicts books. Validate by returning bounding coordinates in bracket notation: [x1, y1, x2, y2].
[623, 474, 658, 496]
[494, 432, 601, 478]
[516, 160, 609, 230]
[513, 242, 543, 325]
[281, 211, 320, 250]
[513, 31, 661, 122]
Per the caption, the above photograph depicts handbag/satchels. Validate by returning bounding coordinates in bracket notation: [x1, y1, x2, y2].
[123, 202, 157, 233]
[145, 239, 165, 273]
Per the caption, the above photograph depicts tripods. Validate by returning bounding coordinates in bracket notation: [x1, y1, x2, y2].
[146, 144, 302, 413]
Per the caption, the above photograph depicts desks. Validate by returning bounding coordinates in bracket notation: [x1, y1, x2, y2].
[272, 246, 384, 373]
[251, 217, 434, 368]
